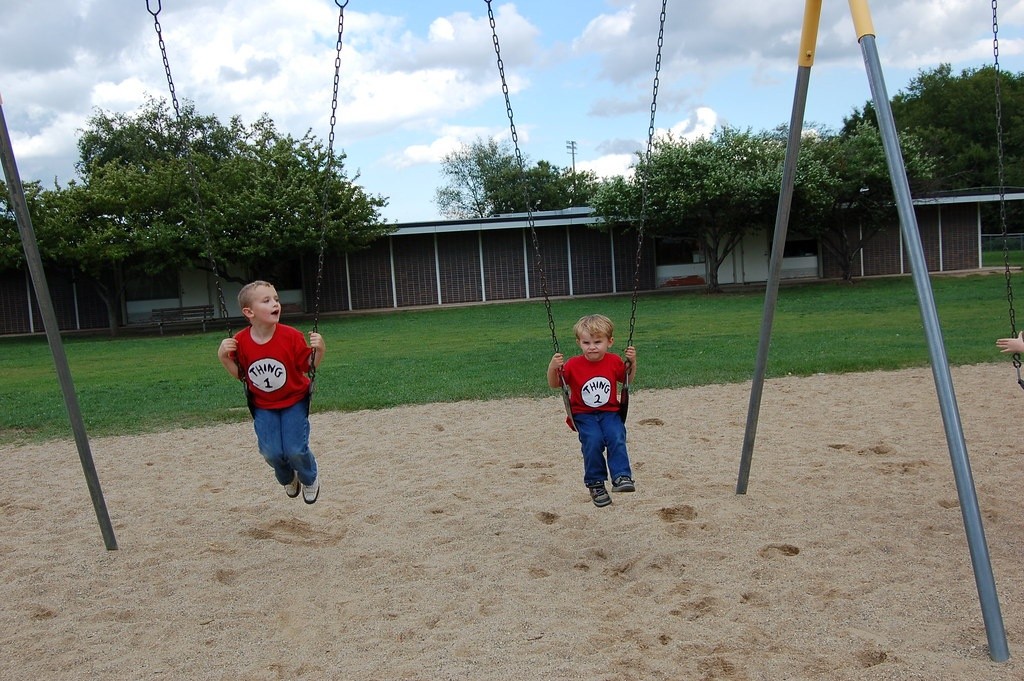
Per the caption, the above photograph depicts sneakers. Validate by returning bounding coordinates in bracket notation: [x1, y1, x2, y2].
[586, 480, 612, 507]
[302, 459, 320, 504]
[284, 470, 301, 498]
[611, 476, 635, 492]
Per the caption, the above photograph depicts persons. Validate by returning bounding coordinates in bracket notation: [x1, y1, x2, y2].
[217, 279, 326, 505]
[547, 313, 637, 507]
[996, 330, 1024, 352]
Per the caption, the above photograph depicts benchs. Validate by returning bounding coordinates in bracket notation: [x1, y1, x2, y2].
[141, 304, 215, 335]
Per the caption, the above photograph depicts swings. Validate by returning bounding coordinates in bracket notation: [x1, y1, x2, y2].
[480, 0, 674, 434]
[142, 0, 353, 420]
